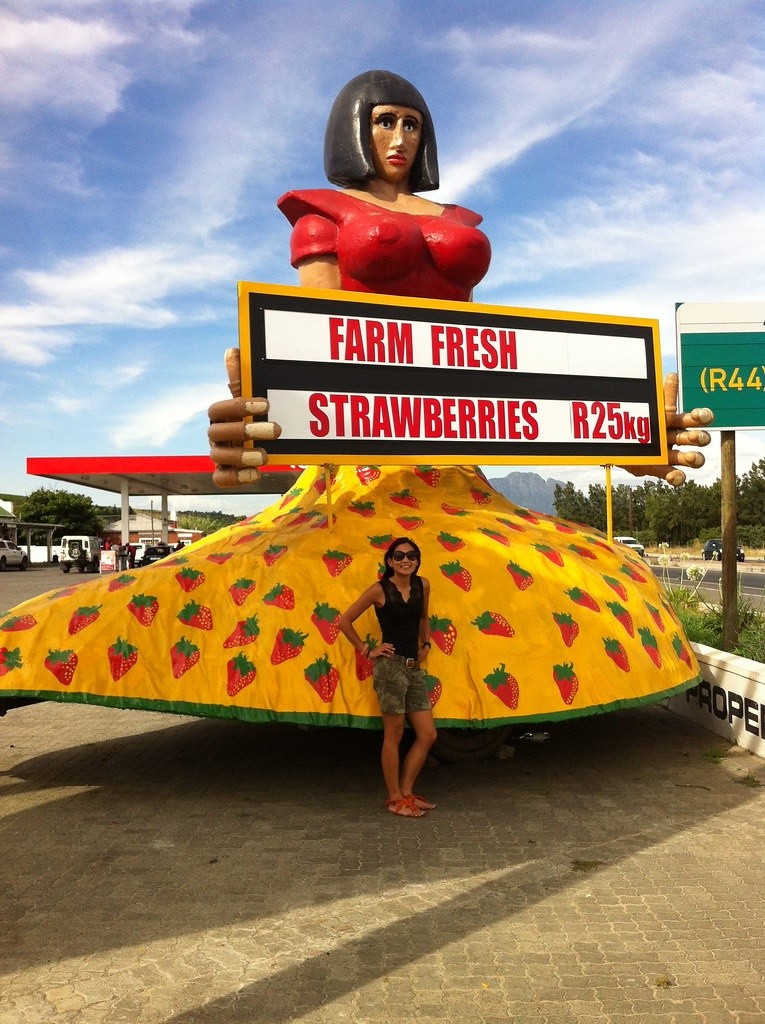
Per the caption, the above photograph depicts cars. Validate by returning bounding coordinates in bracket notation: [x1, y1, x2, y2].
[612, 537, 644, 558]
[0, 539, 29, 571]
[701, 539, 745, 562]
[138, 546, 176, 567]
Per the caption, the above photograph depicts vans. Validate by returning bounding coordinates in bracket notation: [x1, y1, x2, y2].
[58, 536, 103, 573]
[129, 542, 153, 568]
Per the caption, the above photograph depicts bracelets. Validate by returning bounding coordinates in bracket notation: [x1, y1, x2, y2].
[361, 646, 371, 658]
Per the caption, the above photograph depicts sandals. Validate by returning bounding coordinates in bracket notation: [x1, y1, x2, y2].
[386, 794, 437, 818]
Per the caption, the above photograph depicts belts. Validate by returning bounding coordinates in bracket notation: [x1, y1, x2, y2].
[384, 652, 420, 669]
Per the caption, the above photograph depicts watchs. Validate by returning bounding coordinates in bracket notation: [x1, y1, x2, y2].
[422, 642, 431, 649]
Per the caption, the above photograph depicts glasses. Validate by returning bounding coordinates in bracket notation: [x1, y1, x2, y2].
[393, 550, 418, 562]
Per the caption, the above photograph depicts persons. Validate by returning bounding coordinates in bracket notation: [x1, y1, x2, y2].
[125, 544, 136, 569]
[339, 536, 438, 817]
[0, 70, 713, 729]
[174, 540, 185, 552]
[105, 538, 112, 551]
